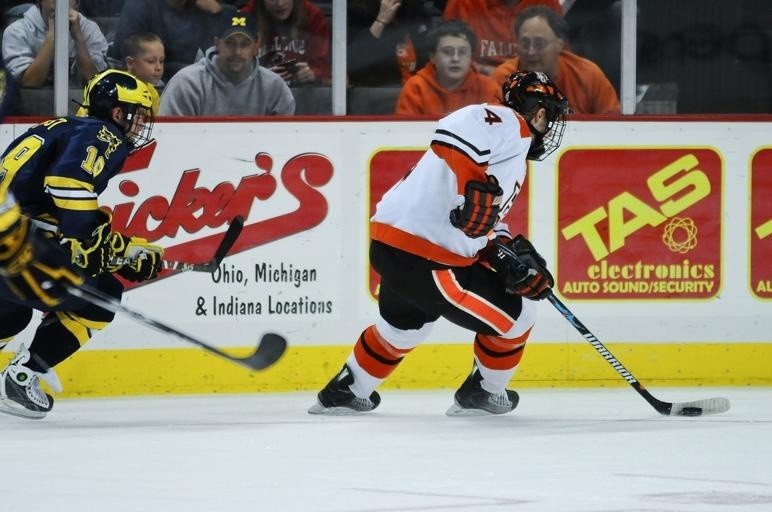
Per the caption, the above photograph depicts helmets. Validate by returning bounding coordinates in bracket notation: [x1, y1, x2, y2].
[83, 68, 153, 142]
[501, 69, 568, 134]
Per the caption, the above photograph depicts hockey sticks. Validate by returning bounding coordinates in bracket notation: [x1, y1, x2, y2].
[67, 283, 287, 369]
[547, 294, 730, 416]
[20, 216, 244, 273]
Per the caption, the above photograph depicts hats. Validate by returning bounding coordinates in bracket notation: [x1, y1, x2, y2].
[214, 9, 259, 43]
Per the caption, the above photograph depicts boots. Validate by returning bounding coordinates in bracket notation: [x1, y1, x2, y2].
[0, 344, 55, 413]
[317, 363, 380, 413]
[455, 358, 520, 414]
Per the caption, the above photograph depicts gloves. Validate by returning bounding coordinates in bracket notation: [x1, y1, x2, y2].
[110, 227, 164, 281]
[67, 208, 113, 277]
[503, 234, 555, 301]
[449, 176, 504, 240]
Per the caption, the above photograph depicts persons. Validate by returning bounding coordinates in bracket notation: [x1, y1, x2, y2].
[1, 68, 163, 411]
[346, 1, 619, 114]
[3, 2, 331, 114]
[318, 69, 575, 414]
